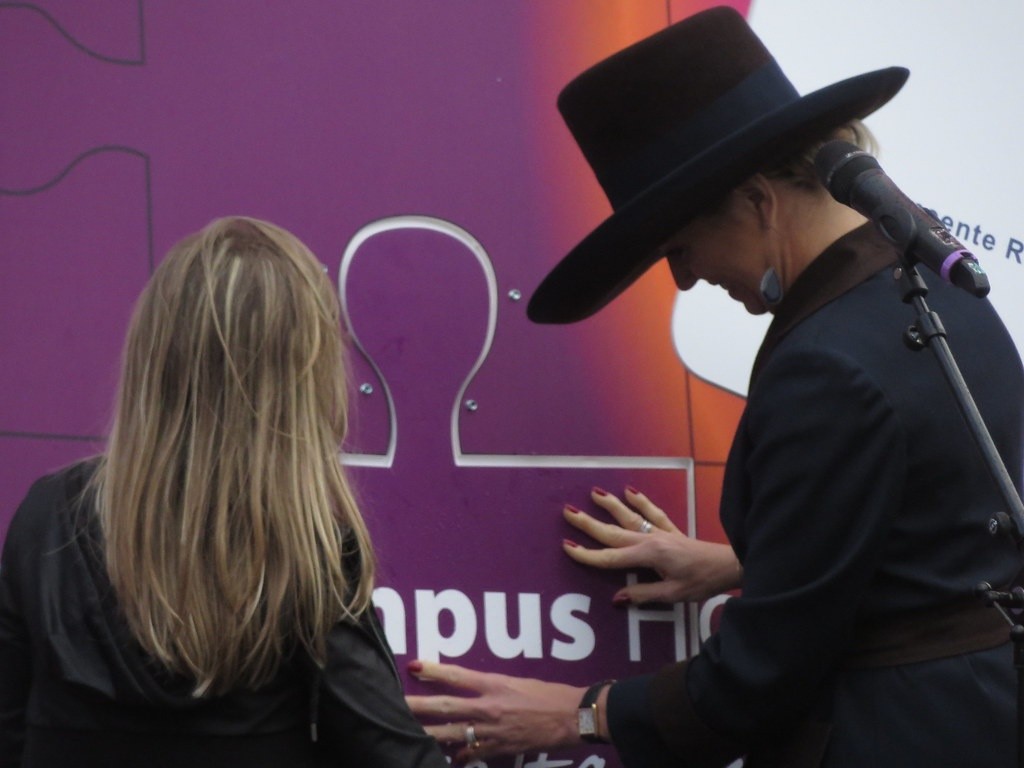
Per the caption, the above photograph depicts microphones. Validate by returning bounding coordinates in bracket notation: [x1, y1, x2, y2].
[814, 139, 991, 298]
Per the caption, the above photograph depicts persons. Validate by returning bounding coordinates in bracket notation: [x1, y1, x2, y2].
[403, 6, 1024, 768]
[0, 216, 450, 768]
[465, 726, 477, 744]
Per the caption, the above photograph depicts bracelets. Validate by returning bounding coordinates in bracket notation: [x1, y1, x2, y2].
[736, 559, 742, 580]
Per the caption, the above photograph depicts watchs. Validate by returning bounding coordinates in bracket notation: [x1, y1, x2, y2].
[577, 680, 611, 744]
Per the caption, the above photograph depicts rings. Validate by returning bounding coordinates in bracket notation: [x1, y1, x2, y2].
[641, 521, 651, 532]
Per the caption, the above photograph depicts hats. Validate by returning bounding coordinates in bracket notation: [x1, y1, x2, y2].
[526, 5, 911, 327]
